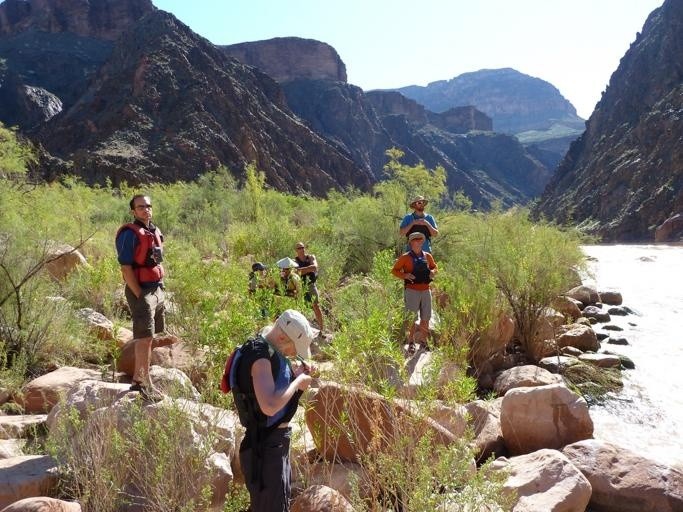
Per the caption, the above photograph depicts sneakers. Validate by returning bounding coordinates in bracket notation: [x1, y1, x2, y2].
[420, 340, 429, 352]
[408, 341, 415, 353]
[318, 330, 326, 338]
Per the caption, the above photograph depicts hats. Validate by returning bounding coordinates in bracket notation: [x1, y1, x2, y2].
[407, 232, 425, 243]
[276, 308, 312, 360]
[275, 257, 299, 269]
[251, 262, 265, 270]
[409, 196, 428, 208]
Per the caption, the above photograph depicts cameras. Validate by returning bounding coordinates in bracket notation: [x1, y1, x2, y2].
[153, 247, 163, 263]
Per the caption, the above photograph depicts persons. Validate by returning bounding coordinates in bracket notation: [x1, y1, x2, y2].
[247, 240, 325, 331]
[391, 232, 439, 353]
[115, 193, 167, 403]
[398, 194, 439, 255]
[219, 308, 314, 512]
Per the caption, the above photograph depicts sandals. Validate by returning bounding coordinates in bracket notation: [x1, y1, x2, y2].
[129, 379, 163, 402]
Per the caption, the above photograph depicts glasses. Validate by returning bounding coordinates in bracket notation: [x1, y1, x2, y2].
[296, 247, 304, 249]
[134, 203, 151, 208]
